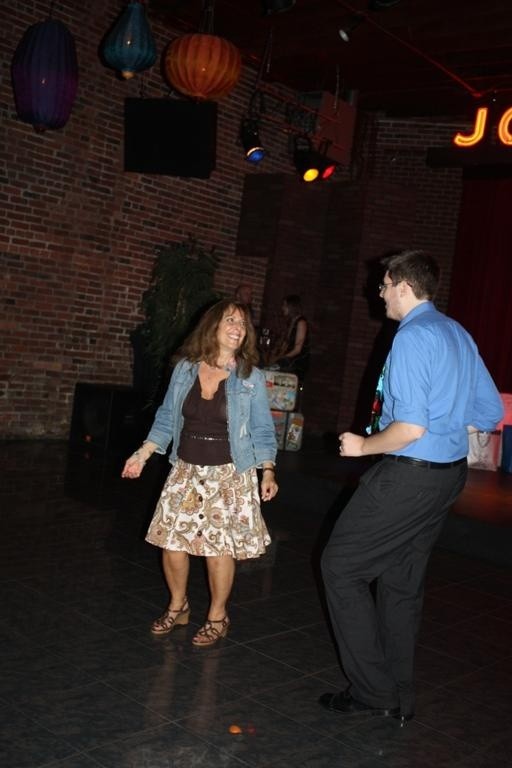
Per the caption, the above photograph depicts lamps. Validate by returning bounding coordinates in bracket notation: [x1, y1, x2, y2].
[236, 114, 339, 187]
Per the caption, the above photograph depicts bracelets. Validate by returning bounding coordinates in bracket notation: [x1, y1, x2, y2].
[132, 452, 147, 466]
[141, 446, 153, 456]
[262, 468, 276, 473]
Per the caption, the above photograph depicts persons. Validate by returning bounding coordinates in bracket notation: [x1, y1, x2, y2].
[272, 292, 311, 389]
[234, 282, 253, 327]
[317, 250, 506, 723]
[121, 299, 278, 647]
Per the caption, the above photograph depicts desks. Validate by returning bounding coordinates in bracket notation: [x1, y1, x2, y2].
[449, 467, 511, 531]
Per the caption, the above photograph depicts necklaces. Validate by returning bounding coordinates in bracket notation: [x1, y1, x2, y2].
[285, 314, 300, 339]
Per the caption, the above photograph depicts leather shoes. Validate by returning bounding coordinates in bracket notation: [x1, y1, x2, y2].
[319, 686, 401, 718]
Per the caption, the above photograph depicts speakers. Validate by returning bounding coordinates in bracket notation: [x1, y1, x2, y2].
[70, 382, 143, 449]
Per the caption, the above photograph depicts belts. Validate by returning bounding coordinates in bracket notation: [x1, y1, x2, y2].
[382, 452, 468, 469]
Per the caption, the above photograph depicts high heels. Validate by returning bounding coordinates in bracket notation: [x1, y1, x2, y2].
[191, 608, 231, 648]
[151, 598, 192, 635]
